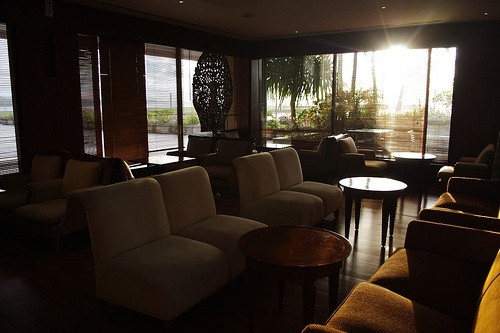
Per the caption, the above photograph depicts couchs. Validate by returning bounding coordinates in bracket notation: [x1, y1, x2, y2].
[0, 152, 112, 255]
[178, 135, 252, 201]
[301, 220, 500, 333]
[417, 176, 500, 231]
[436, 144, 495, 192]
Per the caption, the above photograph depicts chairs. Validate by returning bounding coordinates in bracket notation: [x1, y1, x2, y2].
[80, 178, 230, 333]
[233, 152, 324, 226]
[269, 147, 342, 219]
[300, 133, 388, 179]
[156, 166, 269, 277]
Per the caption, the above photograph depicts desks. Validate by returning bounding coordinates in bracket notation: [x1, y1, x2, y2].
[393, 158, 433, 213]
[338, 176, 408, 246]
[238, 224, 352, 333]
[128, 154, 196, 172]
